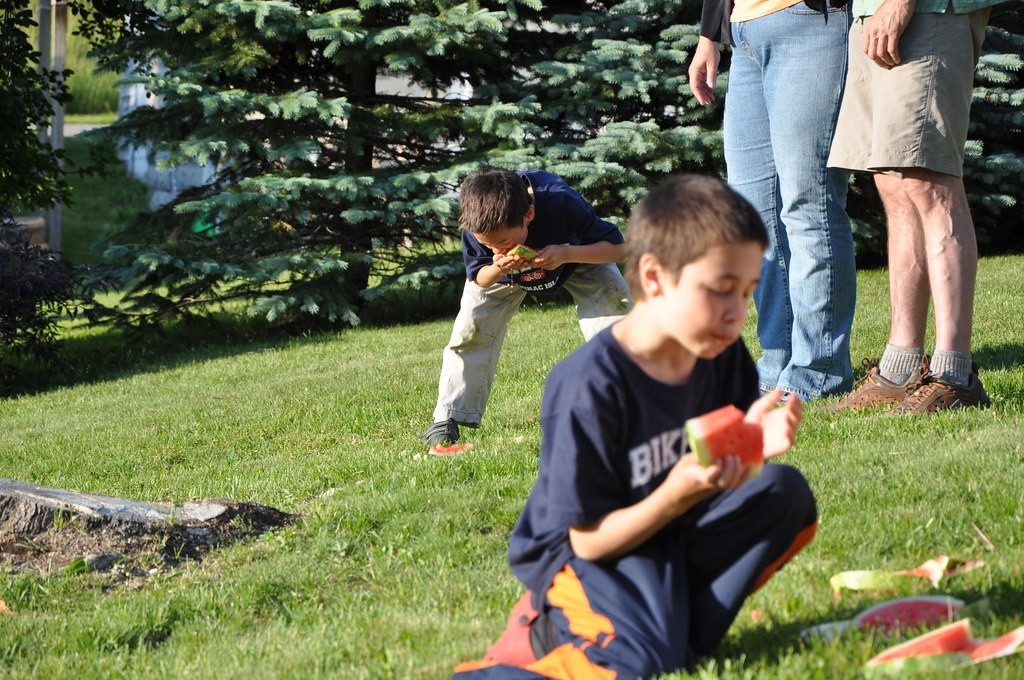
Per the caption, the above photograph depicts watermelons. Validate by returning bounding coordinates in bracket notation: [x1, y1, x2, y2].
[505, 244, 540, 262]
[824, 556, 984, 599]
[685, 404, 765, 476]
[863, 618, 1024, 678]
[848, 594, 964, 636]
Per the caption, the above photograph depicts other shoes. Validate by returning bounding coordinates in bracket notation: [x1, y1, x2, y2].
[418, 418, 460, 452]
[484, 591, 540, 667]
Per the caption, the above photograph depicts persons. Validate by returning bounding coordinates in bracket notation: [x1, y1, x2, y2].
[424, 166, 632, 452]
[688, 0, 857, 404]
[447, 174, 819, 680]
[826, 0, 1002, 416]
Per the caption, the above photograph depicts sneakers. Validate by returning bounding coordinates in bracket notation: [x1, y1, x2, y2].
[887, 356, 990, 418]
[819, 354, 929, 415]
